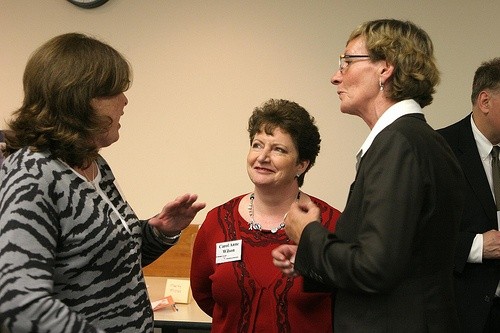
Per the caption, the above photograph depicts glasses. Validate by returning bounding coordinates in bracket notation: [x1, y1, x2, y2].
[339, 55, 369, 69]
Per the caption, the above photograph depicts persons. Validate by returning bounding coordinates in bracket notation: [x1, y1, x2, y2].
[435, 57, 500, 333]
[272, 18, 468, 333]
[0, 32, 206, 333]
[190, 98, 343, 333]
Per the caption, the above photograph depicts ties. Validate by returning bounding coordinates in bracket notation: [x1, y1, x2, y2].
[491, 146, 500, 211]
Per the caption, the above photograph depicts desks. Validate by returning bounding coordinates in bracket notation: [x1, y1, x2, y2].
[144, 276, 213, 333]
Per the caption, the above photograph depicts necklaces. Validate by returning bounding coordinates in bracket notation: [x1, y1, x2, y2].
[249, 188, 301, 233]
[81, 160, 98, 190]
